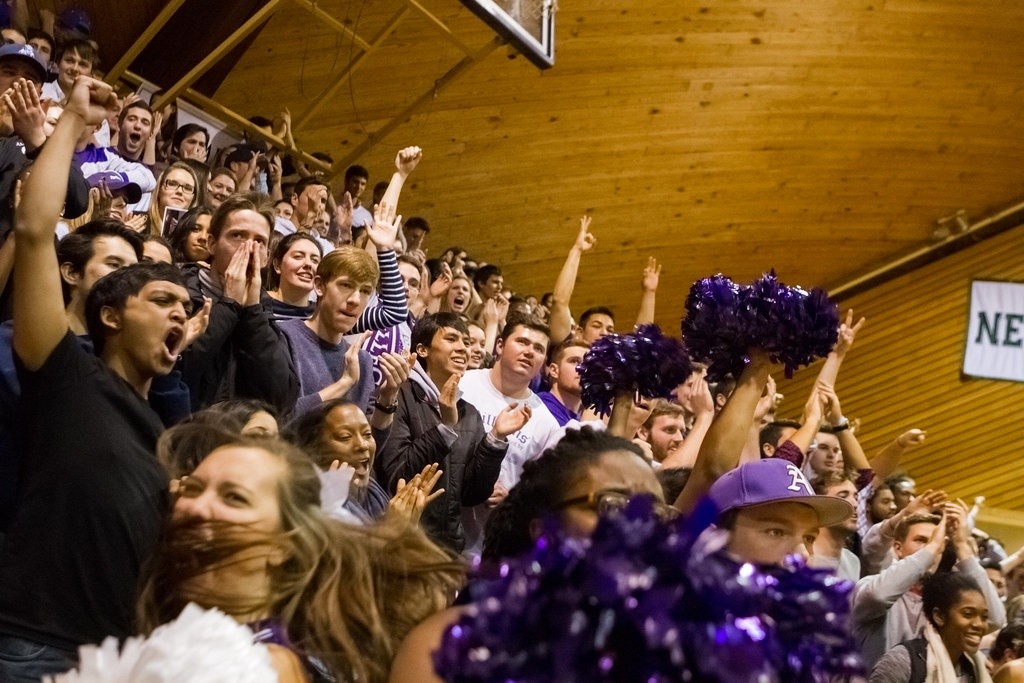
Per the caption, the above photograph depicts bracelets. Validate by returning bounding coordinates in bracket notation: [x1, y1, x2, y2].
[300, 222, 312, 230]
[897, 438, 905, 448]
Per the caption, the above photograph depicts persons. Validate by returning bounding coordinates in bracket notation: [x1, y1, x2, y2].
[0, 0, 1024, 683]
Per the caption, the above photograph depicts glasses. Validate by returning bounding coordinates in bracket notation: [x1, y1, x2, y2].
[163, 179, 197, 195]
[565, 488, 685, 529]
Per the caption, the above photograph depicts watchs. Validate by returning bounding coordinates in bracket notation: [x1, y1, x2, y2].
[375, 396, 398, 413]
[833, 421, 850, 432]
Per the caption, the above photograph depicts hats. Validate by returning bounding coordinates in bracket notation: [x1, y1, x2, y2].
[58, 8, 89, 34]
[0, 44, 49, 80]
[223, 147, 254, 167]
[1004, 594, 1024, 632]
[706, 458, 853, 526]
[86, 171, 143, 204]
